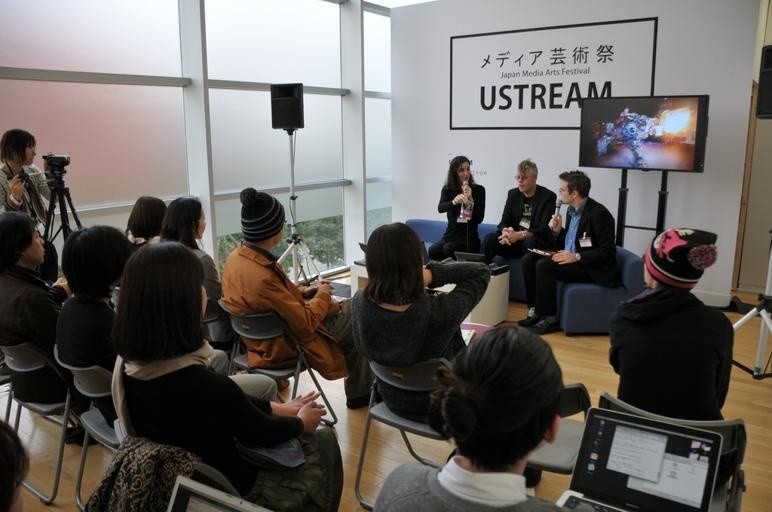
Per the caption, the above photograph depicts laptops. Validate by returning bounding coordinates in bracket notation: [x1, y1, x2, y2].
[555, 406, 724, 512]
[453, 251, 490, 267]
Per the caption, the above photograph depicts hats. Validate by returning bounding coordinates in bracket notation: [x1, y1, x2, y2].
[241, 188, 285, 242]
[645, 226, 717, 291]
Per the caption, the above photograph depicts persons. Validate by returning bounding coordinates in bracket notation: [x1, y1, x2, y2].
[1, 129, 66, 376]
[223, 189, 381, 409]
[111, 244, 343, 512]
[1, 194, 231, 448]
[481, 159, 557, 316]
[373, 323, 571, 512]
[518, 172, 621, 334]
[428, 156, 485, 259]
[610, 229, 735, 421]
[352, 223, 490, 425]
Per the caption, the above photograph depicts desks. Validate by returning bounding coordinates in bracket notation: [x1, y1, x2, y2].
[350, 260, 510, 328]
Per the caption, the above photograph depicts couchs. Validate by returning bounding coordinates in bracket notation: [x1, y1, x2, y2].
[402, 218, 645, 336]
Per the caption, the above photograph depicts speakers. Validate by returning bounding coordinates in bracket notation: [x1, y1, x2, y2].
[270, 83, 304, 134]
[755, 45, 772, 120]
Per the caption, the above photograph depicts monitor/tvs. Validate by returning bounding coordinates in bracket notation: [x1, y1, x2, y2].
[578, 95, 711, 173]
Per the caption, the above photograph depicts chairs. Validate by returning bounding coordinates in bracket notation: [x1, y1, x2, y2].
[111, 287, 121, 314]
[51, 342, 119, 512]
[202, 297, 238, 376]
[526, 417, 587, 474]
[354, 357, 458, 510]
[113, 418, 246, 499]
[0, 342, 84, 504]
[219, 299, 337, 428]
[596, 395, 745, 510]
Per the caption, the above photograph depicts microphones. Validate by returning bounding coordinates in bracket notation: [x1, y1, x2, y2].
[462, 179, 469, 199]
[555, 198, 562, 218]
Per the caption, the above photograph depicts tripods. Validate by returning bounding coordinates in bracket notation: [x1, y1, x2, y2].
[275, 197, 323, 286]
[43, 187, 82, 244]
[732, 293, 772, 380]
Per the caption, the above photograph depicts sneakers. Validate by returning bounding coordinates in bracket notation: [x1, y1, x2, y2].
[518, 314, 536, 326]
[531, 318, 560, 333]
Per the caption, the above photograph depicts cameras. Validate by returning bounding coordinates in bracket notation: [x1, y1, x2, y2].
[42, 154, 71, 179]
